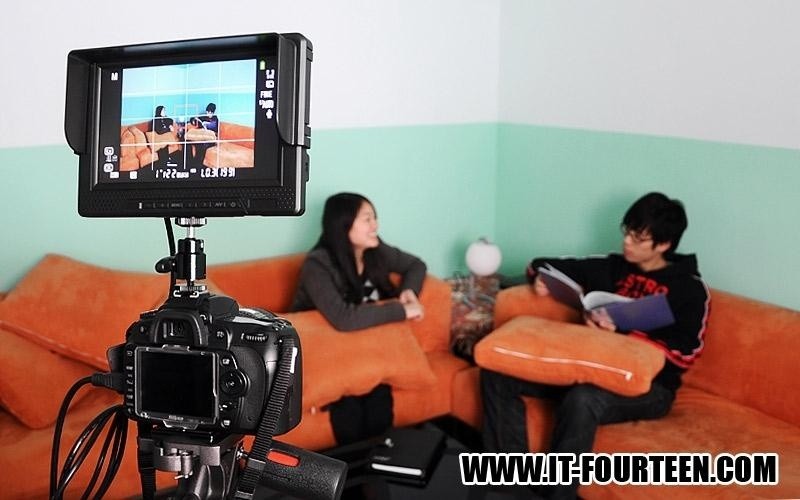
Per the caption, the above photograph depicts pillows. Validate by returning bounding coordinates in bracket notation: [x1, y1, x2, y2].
[146, 131, 177, 152]
[1, 252, 169, 372]
[121, 131, 135, 155]
[273, 300, 440, 410]
[474, 312, 661, 397]
[0, 328, 95, 429]
[184, 128, 216, 141]
[129, 124, 148, 152]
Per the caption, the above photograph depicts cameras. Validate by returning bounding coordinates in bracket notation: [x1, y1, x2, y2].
[64, 32, 348, 500]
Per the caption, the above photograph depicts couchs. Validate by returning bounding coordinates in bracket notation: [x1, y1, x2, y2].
[0, 251, 466, 498]
[120, 120, 180, 170]
[452, 281, 800, 500]
[190, 121, 255, 168]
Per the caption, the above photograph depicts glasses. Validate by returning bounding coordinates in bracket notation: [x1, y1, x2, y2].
[620, 223, 651, 243]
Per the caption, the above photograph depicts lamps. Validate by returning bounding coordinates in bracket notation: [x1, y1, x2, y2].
[466, 239, 503, 276]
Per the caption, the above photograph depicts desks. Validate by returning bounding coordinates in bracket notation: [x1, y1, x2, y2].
[442, 276, 513, 361]
[385, 457, 392, 462]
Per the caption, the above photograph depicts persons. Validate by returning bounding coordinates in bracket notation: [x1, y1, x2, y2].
[149, 106, 175, 171]
[476, 191, 709, 500]
[180, 103, 222, 171]
[277, 194, 429, 444]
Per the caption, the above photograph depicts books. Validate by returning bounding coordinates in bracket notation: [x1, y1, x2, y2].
[538, 261, 675, 334]
[367, 422, 447, 478]
